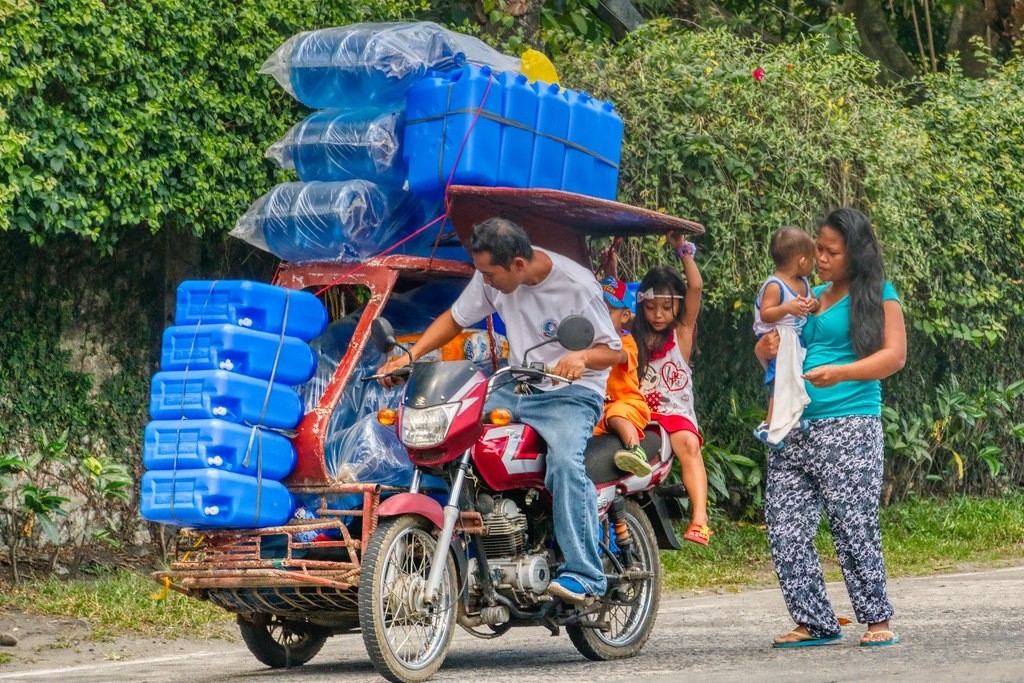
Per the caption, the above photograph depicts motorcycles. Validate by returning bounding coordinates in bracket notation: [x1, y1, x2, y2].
[357, 315, 691, 683]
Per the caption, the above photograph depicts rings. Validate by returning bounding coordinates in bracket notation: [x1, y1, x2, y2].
[567, 373, 574, 377]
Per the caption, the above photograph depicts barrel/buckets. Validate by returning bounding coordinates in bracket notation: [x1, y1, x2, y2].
[563, 89, 597, 196]
[140, 468, 295, 530]
[403, 64, 501, 196]
[590, 98, 623, 201]
[149, 370, 304, 430]
[176, 281, 329, 341]
[530, 80, 570, 190]
[160, 324, 318, 386]
[144, 419, 297, 480]
[494, 71, 537, 188]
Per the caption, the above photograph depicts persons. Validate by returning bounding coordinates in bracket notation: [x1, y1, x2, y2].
[754, 229, 821, 451]
[376, 216, 621, 603]
[754, 208, 908, 648]
[599, 230, 714, 547]
[592, 276, 652, 477]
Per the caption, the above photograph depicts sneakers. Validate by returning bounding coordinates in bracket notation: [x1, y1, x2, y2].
[547, 576, 601, 601]
[613, 436, 653, 478]
[790, 420, 811, 433]
[753, 420, 785, 450]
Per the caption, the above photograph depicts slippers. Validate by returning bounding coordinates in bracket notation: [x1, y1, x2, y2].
[858, 630, 902, 647]
[683, 523, 712, 546]
[772, 631, 844, 649]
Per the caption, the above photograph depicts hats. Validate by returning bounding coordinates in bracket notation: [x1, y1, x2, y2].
[599, 275, 634, 309]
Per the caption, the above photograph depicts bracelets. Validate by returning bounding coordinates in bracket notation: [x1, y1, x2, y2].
[677, 242, 696, 261]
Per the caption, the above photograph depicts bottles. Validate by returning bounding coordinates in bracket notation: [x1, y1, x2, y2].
[267, 23, 521, 260]
[214, 280, 506, 612]
